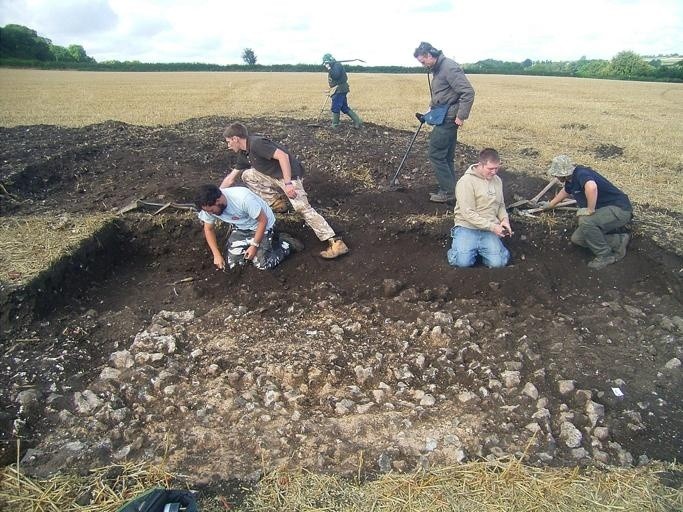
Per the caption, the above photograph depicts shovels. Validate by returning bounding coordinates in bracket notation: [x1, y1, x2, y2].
[115, 198, 199, 215]
[165, 277, 194, 286]
[308, 89, 331, 127]
[507, 199, 579, 212]
[221, 264, 230, 275]
[153, 200, 199, 215]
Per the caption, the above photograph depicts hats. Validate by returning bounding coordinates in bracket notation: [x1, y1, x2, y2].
[546, 156, 575, 178]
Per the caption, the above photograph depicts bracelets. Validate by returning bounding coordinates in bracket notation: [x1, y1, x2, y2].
[251, 242, 259, 248]
[284, 182, 291, 185]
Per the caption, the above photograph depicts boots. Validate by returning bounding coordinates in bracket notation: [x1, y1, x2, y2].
[329, 113, 340, 129]
[347, 110, 362, 130]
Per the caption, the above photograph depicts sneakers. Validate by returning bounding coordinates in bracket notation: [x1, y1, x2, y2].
[270, 202, 287, 213]
[429, 191, 455, 204]
[320, 241, 348, 258]
[588, 233, 630, 269]
[279, 232, 304, 252]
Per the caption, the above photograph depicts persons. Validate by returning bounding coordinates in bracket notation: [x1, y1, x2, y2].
[445, 148, 514, 269]
[412, 41, 475, 203]
[217, 120, 349, 260]
[539, 155, 633, 269]
[321, 54, 362, 128]
[194, 184, 306, 271]
